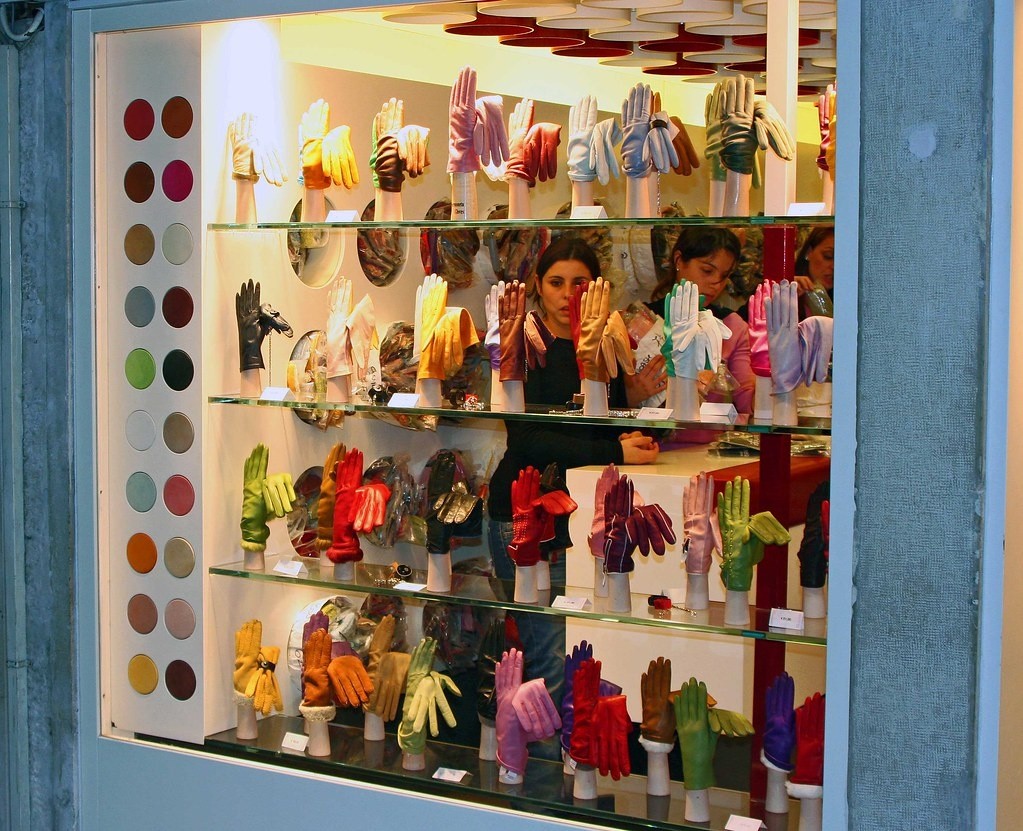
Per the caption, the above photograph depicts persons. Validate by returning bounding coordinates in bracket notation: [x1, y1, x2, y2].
[738, 226, 836, 382]
[633, 226, 757, 417]
[486, 237, 661, 759]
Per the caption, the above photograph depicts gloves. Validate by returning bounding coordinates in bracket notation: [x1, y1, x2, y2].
[765, 279, 834, 396]
[446, 65, 510, 175]
[297, 76, 836, 193]
[228, 112, 289, 188]
[325, 273, 732, 384]
[240, 443, 296, 552]
[233, 620, 284, 716]
[718, 476, 793, 592]
[760, 672, 796, 774]
[682, 470, 722, 576]
[317, 443, 678, 574]
[235, 278, 293, 373]
[299, 610, 756, 790]
[797, 481, 831, 588]
[784, 692, 826, 799]
[747, 279, 780, 377]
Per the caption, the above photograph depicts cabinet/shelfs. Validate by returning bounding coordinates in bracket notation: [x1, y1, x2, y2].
[207, 214, 834, 831]
